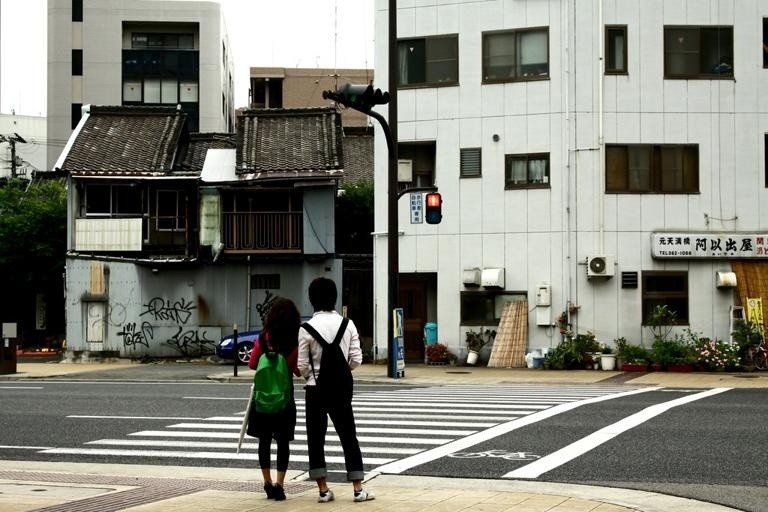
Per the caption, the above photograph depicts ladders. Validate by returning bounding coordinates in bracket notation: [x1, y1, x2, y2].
[729, 305, 752, 361]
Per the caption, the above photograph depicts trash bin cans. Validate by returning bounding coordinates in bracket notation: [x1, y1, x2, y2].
[425, 322, 437, 344]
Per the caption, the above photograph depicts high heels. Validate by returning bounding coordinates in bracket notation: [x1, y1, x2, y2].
[264, 481, 286, 501]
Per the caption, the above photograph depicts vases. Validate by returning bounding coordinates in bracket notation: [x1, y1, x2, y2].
[726, 365, 741, 373]
[710, 365, 725, 372]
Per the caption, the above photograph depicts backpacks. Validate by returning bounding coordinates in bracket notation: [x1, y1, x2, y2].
[302, 316, 354, 410]
[252, 331, 295, 415]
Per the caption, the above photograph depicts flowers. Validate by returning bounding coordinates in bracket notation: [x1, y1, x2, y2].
[426, 342, 450, 360]
[717, 341, 741, 367]
[696, 340, 729, 368]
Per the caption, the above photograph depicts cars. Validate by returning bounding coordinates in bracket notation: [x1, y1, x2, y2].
[216, 315, 313, 364]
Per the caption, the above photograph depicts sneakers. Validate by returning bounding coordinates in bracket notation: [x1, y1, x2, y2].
[354, 488, 375, 502]
[319, 489, 334, 502]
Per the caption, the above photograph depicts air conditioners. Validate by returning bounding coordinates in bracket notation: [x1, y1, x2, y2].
[586, 254, 615, 278]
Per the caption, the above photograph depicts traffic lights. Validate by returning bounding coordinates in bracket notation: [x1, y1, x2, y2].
[336, 83, 389, 108]
[425, 193, 442, 224]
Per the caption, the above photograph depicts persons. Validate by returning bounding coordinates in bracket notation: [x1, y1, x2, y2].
[296, 276, 375, 502]
[245, 297, 303, 501]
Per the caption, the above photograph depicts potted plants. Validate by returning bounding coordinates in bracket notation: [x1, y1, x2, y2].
[732, 318, 762, 372]
[465, 328, 497, 365]
[542, 306, 693, 372]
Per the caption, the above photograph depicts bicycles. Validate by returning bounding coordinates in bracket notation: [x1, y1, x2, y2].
[754, 342, 768, 370]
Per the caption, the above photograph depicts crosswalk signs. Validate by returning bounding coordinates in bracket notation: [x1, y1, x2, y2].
[393, 308, 404, 338]
